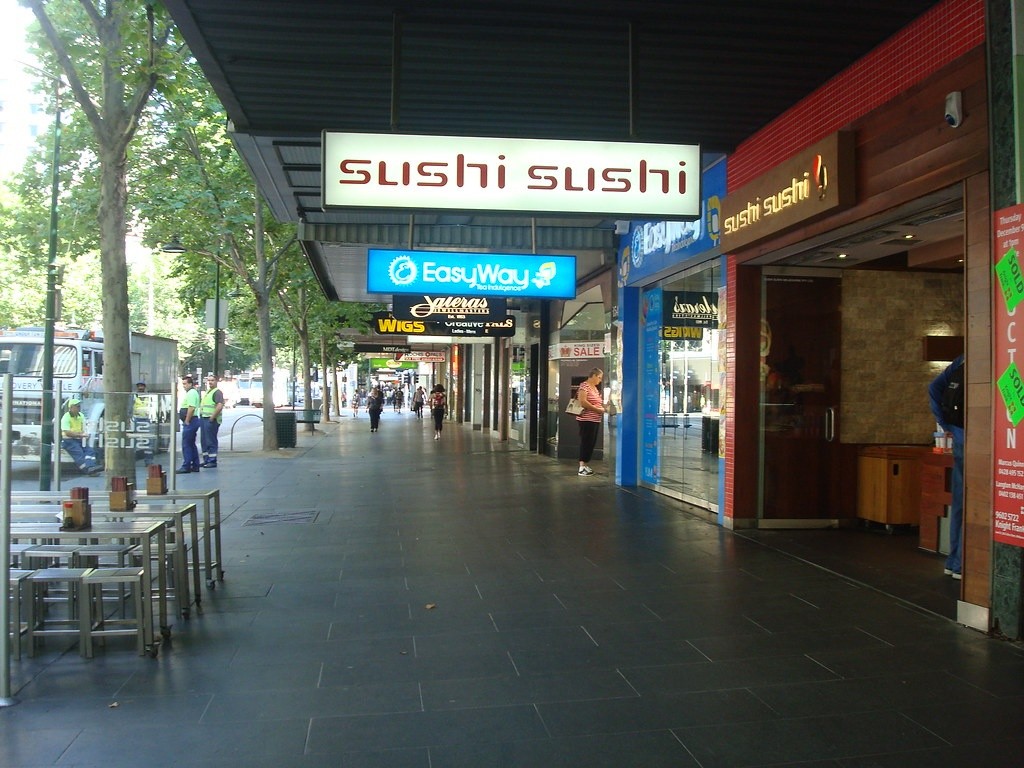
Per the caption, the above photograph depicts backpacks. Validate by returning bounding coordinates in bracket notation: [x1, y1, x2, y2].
[434, 392, 444, 406]
[940, 364, 964, 429]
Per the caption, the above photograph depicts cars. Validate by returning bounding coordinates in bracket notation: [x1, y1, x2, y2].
[295, 386, 319, 403]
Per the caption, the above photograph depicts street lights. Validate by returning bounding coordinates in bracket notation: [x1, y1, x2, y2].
[158, 191, 221, 381]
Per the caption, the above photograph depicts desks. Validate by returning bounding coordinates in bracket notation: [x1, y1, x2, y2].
[10, 503, 202, 620]
[10, 521, 171, 658]
[11, 489, 226, 591]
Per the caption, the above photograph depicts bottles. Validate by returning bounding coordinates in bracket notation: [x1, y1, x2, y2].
[63, 502, 74, 528]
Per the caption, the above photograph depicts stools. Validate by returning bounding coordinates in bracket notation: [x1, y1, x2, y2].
[8, 521, 211, 661]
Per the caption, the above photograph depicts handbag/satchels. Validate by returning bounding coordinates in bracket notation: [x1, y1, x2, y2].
[565, 387, 588, 417]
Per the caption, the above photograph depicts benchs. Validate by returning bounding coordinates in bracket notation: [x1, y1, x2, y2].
[657, 413, 679, 435]
[273, 409, 321, 436]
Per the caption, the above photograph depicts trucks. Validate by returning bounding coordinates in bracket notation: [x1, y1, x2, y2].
[0, 326, 178, 456]
[238, 372, 289, 407]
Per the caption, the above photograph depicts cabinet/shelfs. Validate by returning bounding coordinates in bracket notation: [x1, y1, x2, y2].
[855, 444, 927, 535]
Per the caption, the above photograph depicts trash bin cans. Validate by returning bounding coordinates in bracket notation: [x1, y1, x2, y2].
[312, 398, 322, 409]
[275, 413, 296, 448]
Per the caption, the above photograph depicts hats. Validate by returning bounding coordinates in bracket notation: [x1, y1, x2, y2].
[68, 400, 80, 407]
[136, 381, 145, 385]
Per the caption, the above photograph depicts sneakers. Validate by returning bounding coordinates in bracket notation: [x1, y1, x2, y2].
[578, 467, 595, 476]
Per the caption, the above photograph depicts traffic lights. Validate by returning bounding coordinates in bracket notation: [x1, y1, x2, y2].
[414, 372, 419, 385]
[404, 372, 409, 383]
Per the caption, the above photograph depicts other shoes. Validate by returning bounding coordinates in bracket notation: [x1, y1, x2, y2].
[192, 466, 199, 472]
[944, 567, 953, 574]
[176, 468, 190, 473]
[371, 429, 373, 432]
[434, 434, 440, 440]
[952, 570, 961, 579]
[204, 462, 217, 467]
[200, 461, 207, 467]
[374, 429, 377, 432]
[89, 465, 105, 474]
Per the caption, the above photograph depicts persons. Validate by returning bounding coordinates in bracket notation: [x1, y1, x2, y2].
[929, 352, 965, 580]
[512, 388, 518, 422]
[61, 399, 103, 477]
[342, 391, 346, 407]
[351, 389, 361, 418]
[376, 384, 404, 414]
[128, 382, 154, 467]
[430, 384, 446, 440]
[520, 379, 524, 393]
[413, 384, 436, 420]
[366, 388, 384, 432]
[199, 375, 224, 468]
[176, 377, 200, 474]
[359, 387, 373, 407]
[576, 368, 606, 476]
